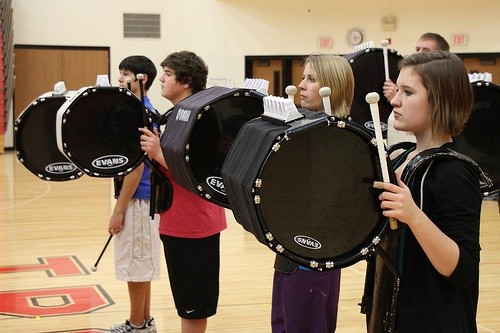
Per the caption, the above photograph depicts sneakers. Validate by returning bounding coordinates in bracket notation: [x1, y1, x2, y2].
[111, 319, 149, 333]
[145, 316, 156, 333]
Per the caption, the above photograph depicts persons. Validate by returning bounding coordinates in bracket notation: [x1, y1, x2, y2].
[271, 55, 354, 333]
[416, 33, 450, 53]
[139, 51, 228, 333]
[360, 51, 493, 333]
[107, 56, 162, 333]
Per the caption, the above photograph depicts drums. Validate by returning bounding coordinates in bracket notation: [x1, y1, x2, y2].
[222, 108, 392, 269]
[13, 92, 84, 182]
[159, 86, 275, 209]
[443, 80, 500, 203]
[56, 86, 153, 178]
[342, 47, 408, 138]
